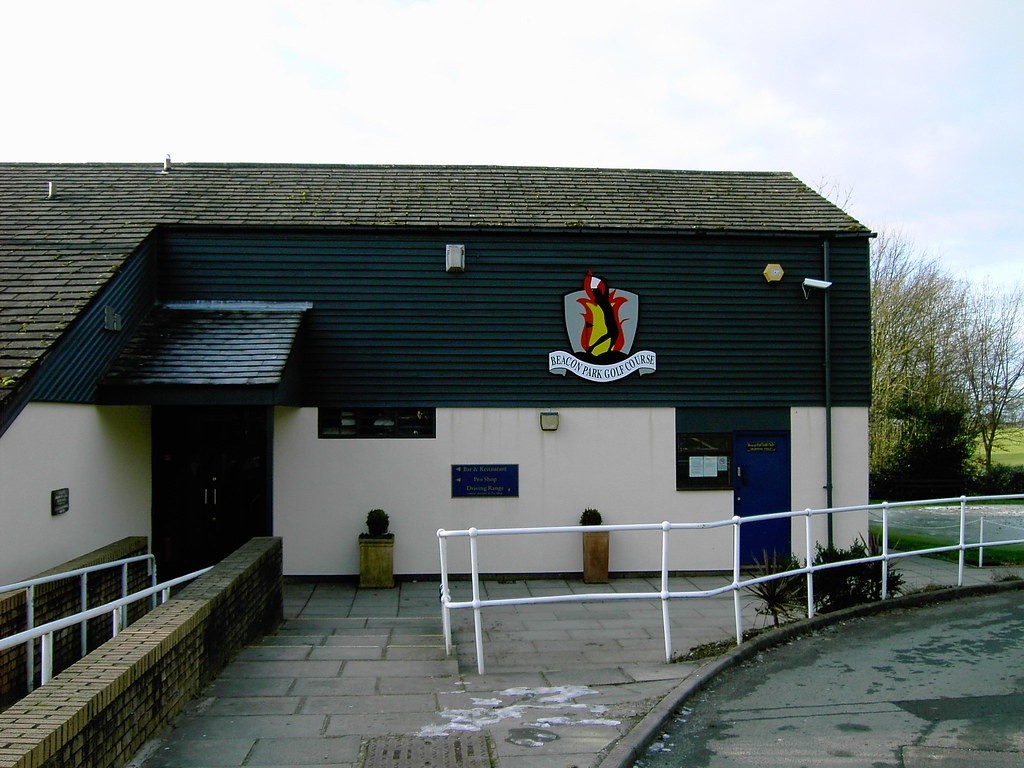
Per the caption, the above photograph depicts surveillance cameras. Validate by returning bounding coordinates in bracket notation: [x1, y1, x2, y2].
[804, 278, 832, 289]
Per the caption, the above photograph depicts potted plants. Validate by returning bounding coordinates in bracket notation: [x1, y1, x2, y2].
[580, 508, 610, 584]
[356, 508, 395, 588]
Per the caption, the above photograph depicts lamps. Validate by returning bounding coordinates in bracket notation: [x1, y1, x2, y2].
[540, 411, 559, 431]
